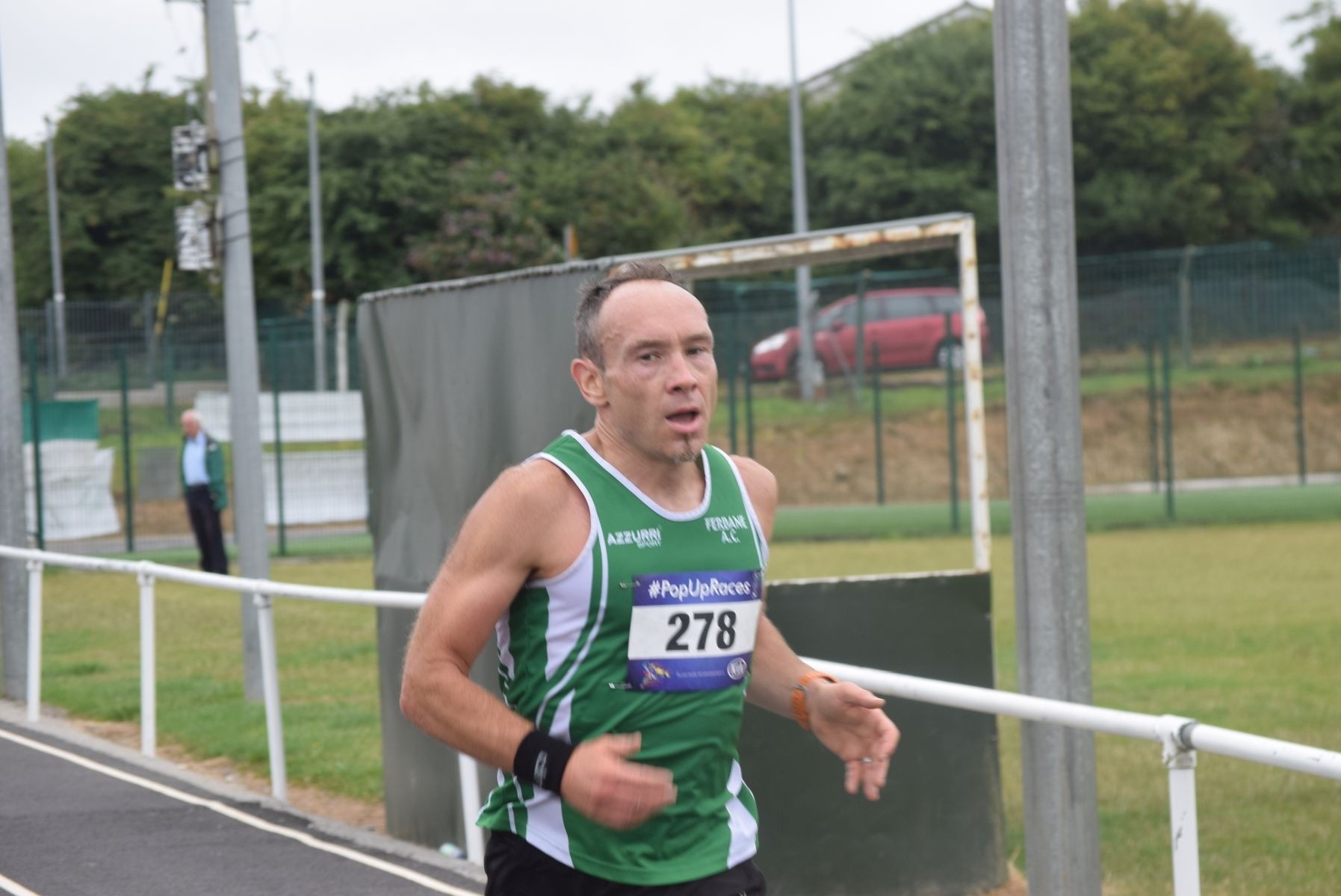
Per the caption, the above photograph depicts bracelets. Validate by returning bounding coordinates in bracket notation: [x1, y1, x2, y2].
[790, 670, 839, 733]
[512, 725, 577, 798]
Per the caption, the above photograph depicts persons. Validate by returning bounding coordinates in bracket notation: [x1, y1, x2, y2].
[179, 408, 231, 574]
[396, 257, 906, 896]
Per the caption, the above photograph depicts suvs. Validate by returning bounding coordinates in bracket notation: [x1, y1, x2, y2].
[749, 289, 992, 381]
